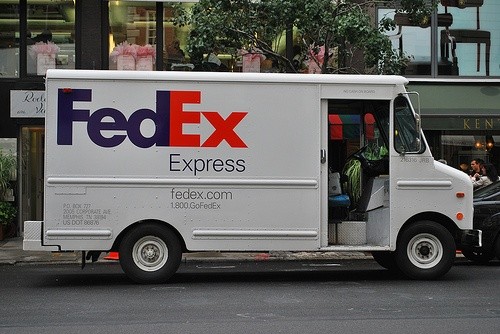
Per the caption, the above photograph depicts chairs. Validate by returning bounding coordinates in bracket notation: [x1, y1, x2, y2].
[328, 194, 350, 222]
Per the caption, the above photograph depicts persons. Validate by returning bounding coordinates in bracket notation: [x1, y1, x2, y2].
[466, 159, 486, 181]
[454, 158, 471, 172]
[470, 163, 500, 187]
[437, 159, 448, 166]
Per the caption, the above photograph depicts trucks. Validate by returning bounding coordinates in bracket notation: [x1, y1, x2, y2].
[23, 68, 474, 285]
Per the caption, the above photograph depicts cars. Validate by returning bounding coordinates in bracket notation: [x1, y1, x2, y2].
[460, 180, 500, 264]
